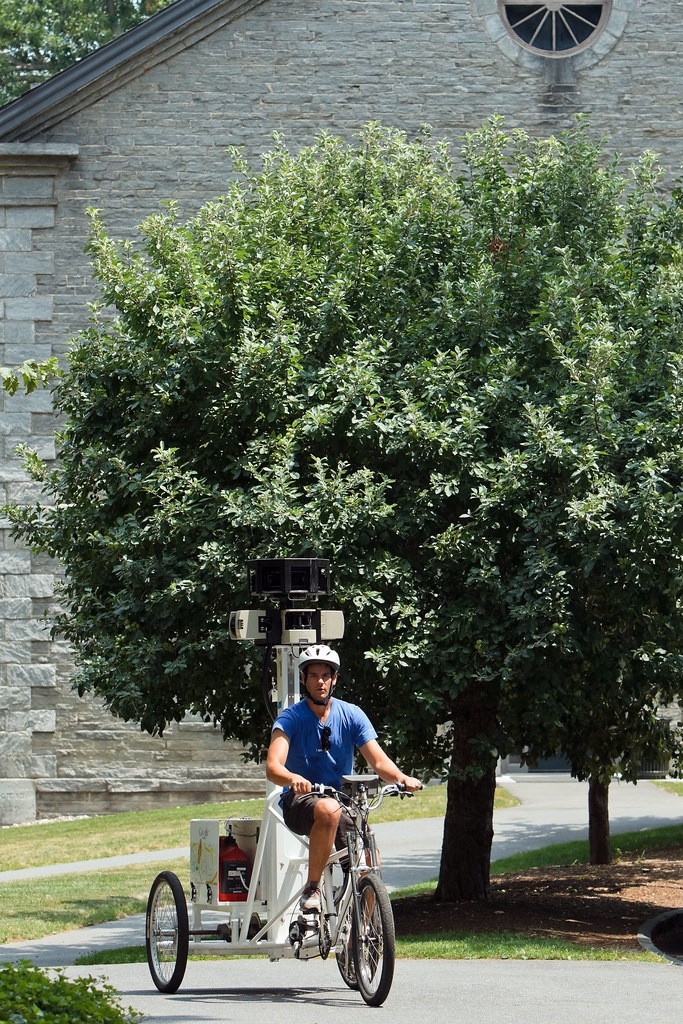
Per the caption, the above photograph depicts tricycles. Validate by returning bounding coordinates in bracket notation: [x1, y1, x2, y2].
[145, 784, 425, 1008]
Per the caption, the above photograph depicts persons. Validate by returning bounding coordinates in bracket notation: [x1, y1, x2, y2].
[266, 645, 421, 981]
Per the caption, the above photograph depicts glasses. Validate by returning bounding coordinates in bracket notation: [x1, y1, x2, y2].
[322, 726, 331, 752]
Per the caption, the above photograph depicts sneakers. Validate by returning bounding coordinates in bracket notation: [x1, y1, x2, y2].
[300, 886, 321, 913]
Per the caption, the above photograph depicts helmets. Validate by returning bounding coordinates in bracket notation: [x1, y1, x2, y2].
[298, 645, 340, 672]
